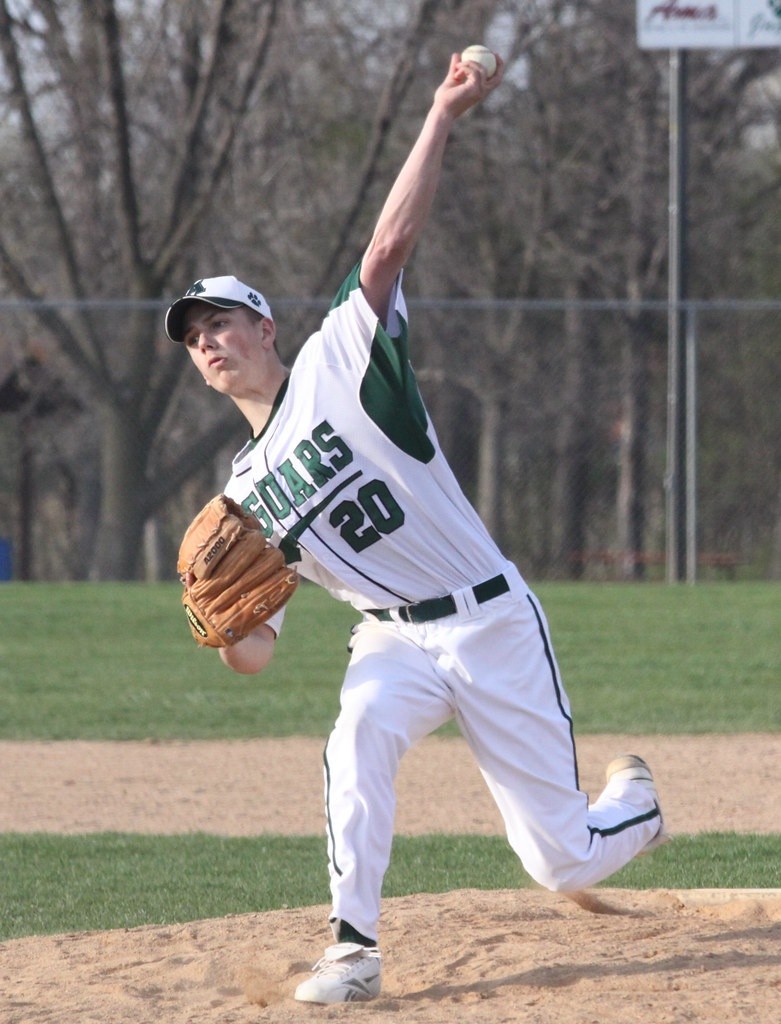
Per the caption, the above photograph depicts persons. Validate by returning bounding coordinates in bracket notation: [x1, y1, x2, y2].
[166, 46, 662, 1007]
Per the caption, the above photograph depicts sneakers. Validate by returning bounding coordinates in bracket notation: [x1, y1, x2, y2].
[294, 942, 382, 1005]
[606, 754, 663, 858]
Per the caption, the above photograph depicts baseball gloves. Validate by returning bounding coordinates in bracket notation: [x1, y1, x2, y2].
[176, 492, 299, 649]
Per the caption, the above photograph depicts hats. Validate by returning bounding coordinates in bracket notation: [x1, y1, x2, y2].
[164, 275, 274, 345]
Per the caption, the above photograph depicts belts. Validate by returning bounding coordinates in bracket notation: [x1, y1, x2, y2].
[366, 574, 509, 624]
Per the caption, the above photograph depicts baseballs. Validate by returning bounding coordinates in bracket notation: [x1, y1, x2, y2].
[461, 44, 497, 80]
